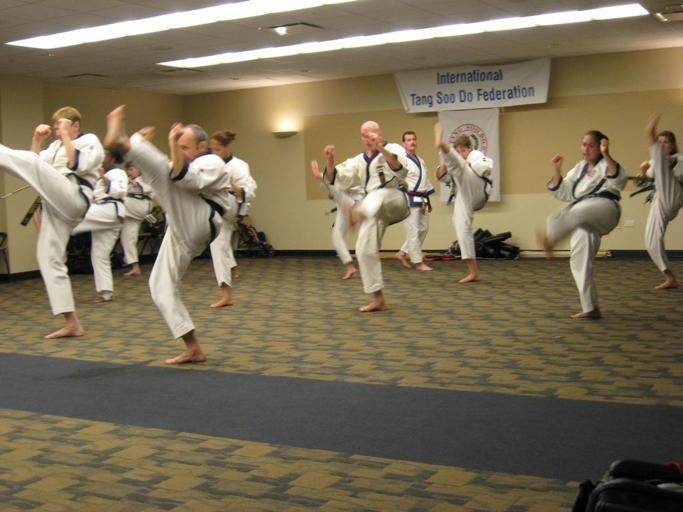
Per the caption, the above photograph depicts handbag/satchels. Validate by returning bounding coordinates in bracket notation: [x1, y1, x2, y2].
[571, 460, 683, 512]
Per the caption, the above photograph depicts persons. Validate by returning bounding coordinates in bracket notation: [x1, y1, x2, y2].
[104, 104, 230, 365]
[310, 159, 365, 281]
[395, 131, 434, 273]
[627, 115, 682, 292]
[34, 147, 126, 305]
[0, 106, 104, 340]
[318, 119, 412, 314]
[209, 129, 257, 309]
[533, 131, 630, 320]
[119, 160, 154, 276]
[435, 122, 494, 285]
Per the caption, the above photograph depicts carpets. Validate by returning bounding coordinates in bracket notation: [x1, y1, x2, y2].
[0, 352, 681, 488]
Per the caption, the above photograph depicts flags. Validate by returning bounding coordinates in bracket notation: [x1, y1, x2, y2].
[436, 106, 502, 205]
